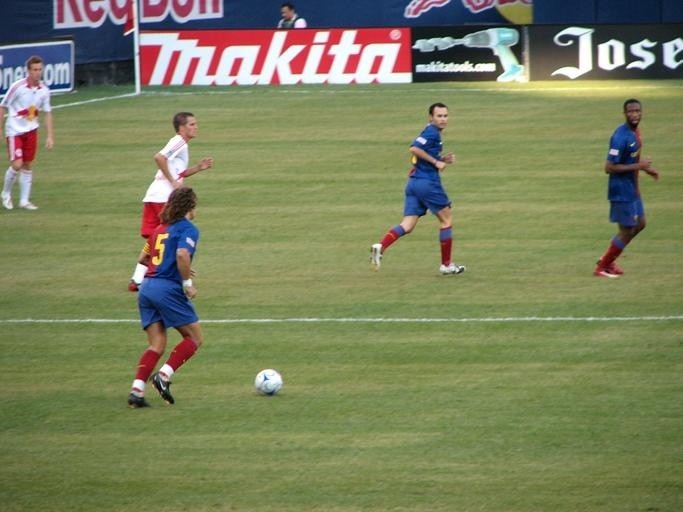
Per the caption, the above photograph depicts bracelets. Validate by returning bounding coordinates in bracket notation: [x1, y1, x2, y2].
[182, 279, 192, 287]
[433, 159, 438, 165]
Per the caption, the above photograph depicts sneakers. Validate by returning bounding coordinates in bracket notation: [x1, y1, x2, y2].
[440, 262, 466, 275]
[128, 279, 143, 292]
[1, 190, 14, 210]
[594, 266, 621, 278]
[368, 243, 384, 272]
[17, 200, 38, 211]
[127, 392, 150, 408]
[149, 373, 175, 405]
[597, 258, 625, 275]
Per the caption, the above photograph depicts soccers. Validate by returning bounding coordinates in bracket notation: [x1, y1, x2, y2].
[255, 369, 283, 395]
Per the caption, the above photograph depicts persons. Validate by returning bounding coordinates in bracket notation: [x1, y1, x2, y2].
[368, 102, 468, 275]
[1, 55, 56, 211]
[127, 188, 205, 408]
[592, 99, 661, 278]
[276, 3, 308, 28]
[128, 111, 216, 292]
